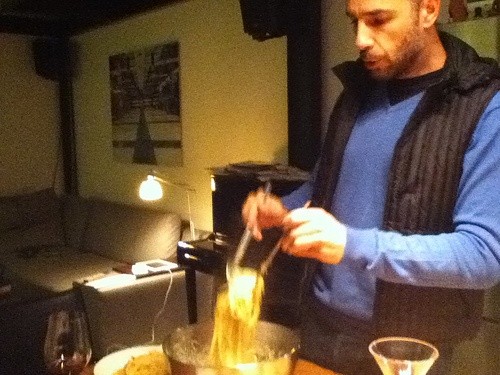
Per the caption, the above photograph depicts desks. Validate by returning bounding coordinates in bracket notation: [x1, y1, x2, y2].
[177, 239, 296, 323]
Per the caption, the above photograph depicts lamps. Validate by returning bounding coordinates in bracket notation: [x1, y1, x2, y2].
[139, 169, 198, 241]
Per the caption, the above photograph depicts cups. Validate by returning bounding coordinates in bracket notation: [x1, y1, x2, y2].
[42, 310, 92, 375]
[368, 336, 439, 375]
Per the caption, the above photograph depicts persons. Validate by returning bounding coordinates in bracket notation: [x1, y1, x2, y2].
[241, 0, 500, 375]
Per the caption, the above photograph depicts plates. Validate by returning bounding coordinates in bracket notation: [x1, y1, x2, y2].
[93, 344, 164, 375]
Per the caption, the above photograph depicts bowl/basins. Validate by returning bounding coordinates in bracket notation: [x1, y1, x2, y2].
[161, 318, 300, 375]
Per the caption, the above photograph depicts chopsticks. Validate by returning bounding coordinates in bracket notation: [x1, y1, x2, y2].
[231, 179, 313, 275]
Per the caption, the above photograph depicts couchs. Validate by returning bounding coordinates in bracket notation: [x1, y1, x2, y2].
[0, 188, 214, 363]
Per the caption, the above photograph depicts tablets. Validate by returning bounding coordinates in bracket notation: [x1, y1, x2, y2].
[140, 260, 179, 274]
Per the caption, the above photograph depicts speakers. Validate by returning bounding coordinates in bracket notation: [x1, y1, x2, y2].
[32, 39, 64, 81]
[239, 0, 292, 40]
